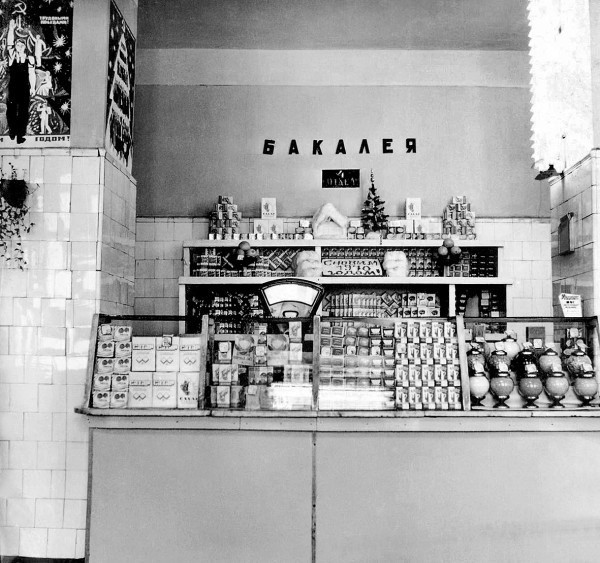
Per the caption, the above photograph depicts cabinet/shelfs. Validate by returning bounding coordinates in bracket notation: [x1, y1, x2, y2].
[177, 241, 513, 321]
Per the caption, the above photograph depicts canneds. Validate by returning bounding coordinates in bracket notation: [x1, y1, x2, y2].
[93, 160, 600, 427]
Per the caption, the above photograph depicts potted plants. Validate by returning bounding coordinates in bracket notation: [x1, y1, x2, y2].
[0, 162, 41, 275]
